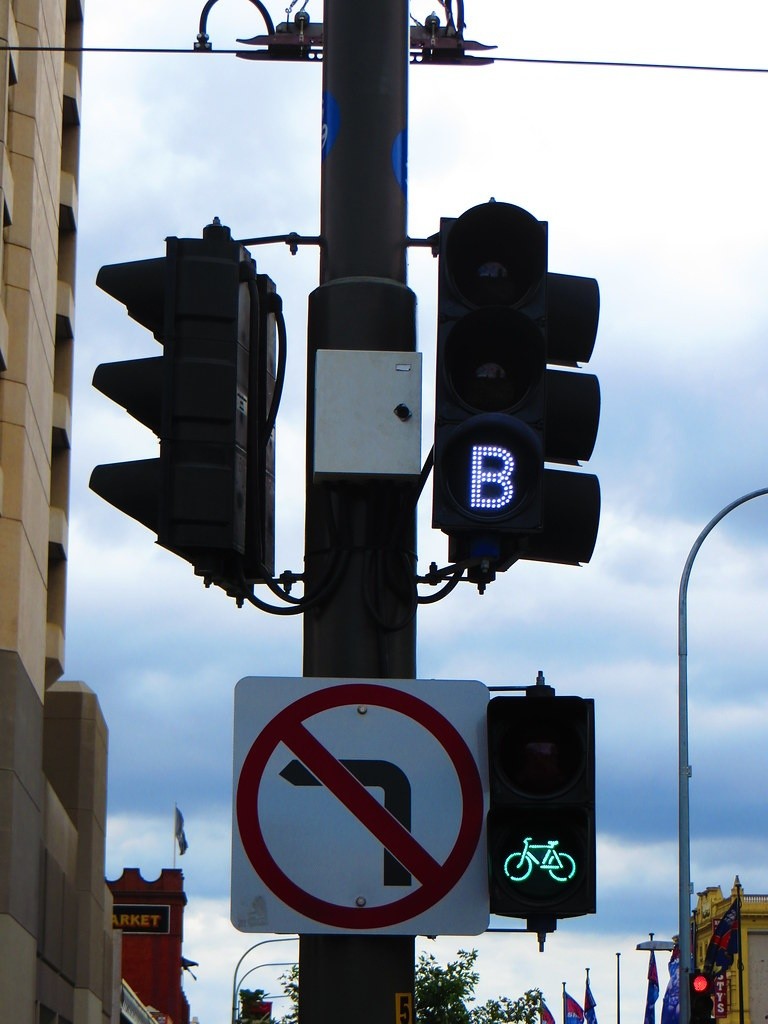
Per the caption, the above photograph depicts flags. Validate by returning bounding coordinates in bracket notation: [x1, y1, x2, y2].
[563, 992, 584, 1024]
[704, 899, 740, 977]
[644, 950, 659, 1024]
[661, 932, 694, 1024]
[175, 809, 188, 854]
[541, 1002, 555, 1024]
[583, 981, 598, 1024]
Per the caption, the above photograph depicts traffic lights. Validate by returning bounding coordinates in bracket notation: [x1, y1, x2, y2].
[80, 229, 274, 593]
[486, 695, 599, 923]
[432, 200, 601, 585]
[690, 973, 712, 1021]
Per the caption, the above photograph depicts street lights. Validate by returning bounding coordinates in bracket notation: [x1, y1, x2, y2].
[638, 941, 678, 954]
